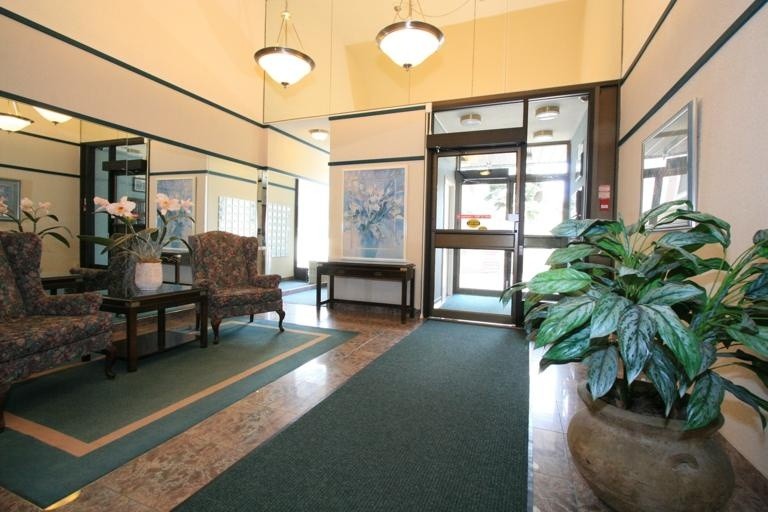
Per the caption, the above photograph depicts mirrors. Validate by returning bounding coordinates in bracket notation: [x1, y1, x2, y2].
[0, 95, 148, 278]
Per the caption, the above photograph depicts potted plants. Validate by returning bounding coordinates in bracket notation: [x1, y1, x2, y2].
[501, 200, 768, 512]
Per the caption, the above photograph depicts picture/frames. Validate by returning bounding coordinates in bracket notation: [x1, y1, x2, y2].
[154, 177, 196, 251]
[132, 177, 145, 192]
[0, 178, 21, 222]
[638, 100, 696, 232]
[340, 165, 408, 263]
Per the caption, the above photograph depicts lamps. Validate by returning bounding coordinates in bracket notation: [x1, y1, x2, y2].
[310, 129, 328, 141]
[254, 0, 316, 89]
[376, 0, 446, 71]
[460, 114, 481, 127]
[534, 129, 553, 141]
[0, 100, 73, 134]
[535, 106, 560, 121]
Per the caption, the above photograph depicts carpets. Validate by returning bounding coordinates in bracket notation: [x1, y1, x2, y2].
[277, 279, 328, 307]
[170, 293, 528, 512]
[0, 317, 361, 509]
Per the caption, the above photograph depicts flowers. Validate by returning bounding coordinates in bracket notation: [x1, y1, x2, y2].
[2, 197, 74, 248]
[76, 193, 195, 262]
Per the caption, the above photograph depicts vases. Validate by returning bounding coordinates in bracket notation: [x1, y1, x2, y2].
[133, 263, 164, 291]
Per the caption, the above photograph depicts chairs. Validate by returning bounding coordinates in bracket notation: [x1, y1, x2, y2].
[187, 231, 285, 345]
[0, 232, 117, 431]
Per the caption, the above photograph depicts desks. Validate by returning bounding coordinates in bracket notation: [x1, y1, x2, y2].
[90, 284, 208, 372]
[316, 262, 416, 324]
